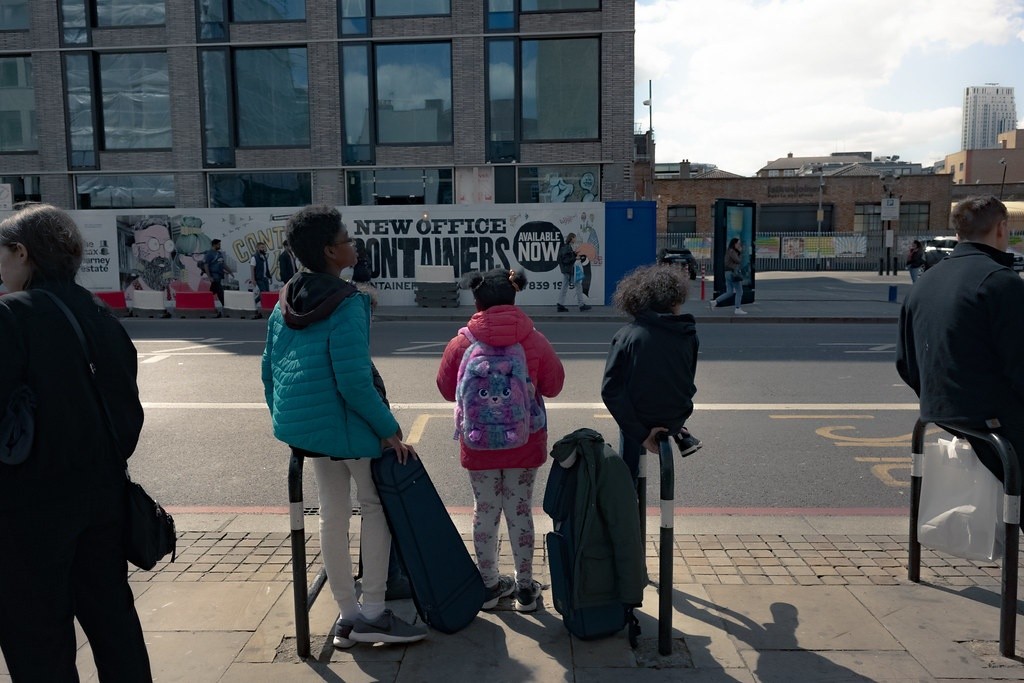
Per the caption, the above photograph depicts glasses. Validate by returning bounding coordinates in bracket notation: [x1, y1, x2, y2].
[333, 238, 352, 248]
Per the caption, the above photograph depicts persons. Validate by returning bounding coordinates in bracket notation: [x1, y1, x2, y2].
[601, 263, 702, 507]
[709, 238, 748, 315]
[276, 239, 298, 287]
[250, 242, 272, 307]
[579, 211, 597, 232]
[204, 239, 235, 310]
[907, 240, 924, 283]
[557, 232, 592, 312]
[436, 267, 565, 613]
[260, 205, 429, 648]
[341, 237, 372, 316]
[895, 195, 1024, 533]
[0, 204, 153, 683]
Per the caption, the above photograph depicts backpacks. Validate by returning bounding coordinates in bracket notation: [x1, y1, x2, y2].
[452, 327, 545, 451]
[351, 254, 372, 283]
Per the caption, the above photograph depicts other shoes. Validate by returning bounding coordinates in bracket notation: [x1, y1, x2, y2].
[557, 303, 569, 312]
[580, 303, 592, 312]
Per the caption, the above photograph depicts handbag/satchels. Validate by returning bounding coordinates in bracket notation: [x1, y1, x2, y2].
[731, 274, 744, 282]
[917, 430, 1003, 563]
[116, 468, 176, 571]
[574, 260, 585, 282]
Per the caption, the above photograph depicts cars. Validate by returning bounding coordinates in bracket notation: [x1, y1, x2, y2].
[916, 235, 1024, 276]
[658, 248, 698, 280]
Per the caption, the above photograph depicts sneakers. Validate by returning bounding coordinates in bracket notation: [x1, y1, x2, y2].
[735, 308, 747, 315]
[673, 427, 703, 458]
[710, 301, 716, 312]
